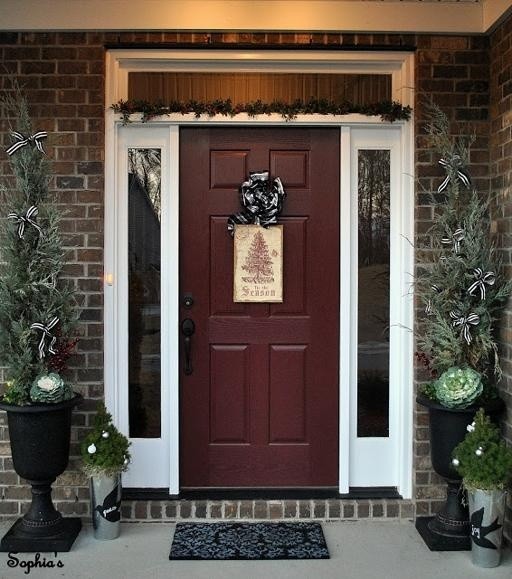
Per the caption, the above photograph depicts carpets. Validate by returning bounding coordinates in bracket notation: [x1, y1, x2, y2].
[169, 522, 330, 560]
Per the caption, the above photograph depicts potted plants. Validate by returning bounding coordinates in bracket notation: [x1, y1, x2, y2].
[0, 60, 82, 553]
[450, 407, 512, 568]
[79, 399, 133, 541]
[396, 83, 512, 551]
